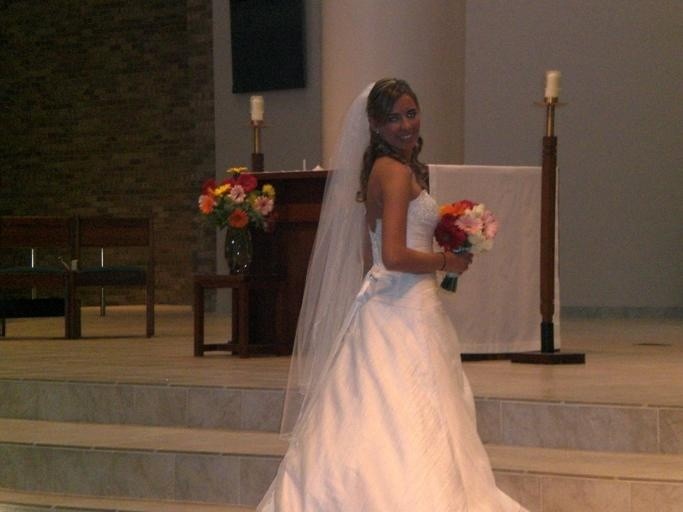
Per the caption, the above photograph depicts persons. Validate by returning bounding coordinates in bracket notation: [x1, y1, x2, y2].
[254, 77, 534, 512]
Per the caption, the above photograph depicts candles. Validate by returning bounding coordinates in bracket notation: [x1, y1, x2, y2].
[544, 70, 561, 98]
[249, 96, 264, 122]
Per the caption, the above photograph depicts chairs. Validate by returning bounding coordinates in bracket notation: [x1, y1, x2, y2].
[1, 209, 158, 340]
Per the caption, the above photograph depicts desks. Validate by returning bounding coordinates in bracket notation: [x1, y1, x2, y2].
[193, 275, 291, 360]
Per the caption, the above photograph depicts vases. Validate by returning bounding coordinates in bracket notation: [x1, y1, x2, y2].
[225, 224, 254, 274]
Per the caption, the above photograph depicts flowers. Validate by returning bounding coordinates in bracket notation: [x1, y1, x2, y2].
[195, 165, 279, 256]
[434, 198, 497, 292]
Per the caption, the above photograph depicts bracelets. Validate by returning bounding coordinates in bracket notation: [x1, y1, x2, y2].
[441, 251, 448, 270]
